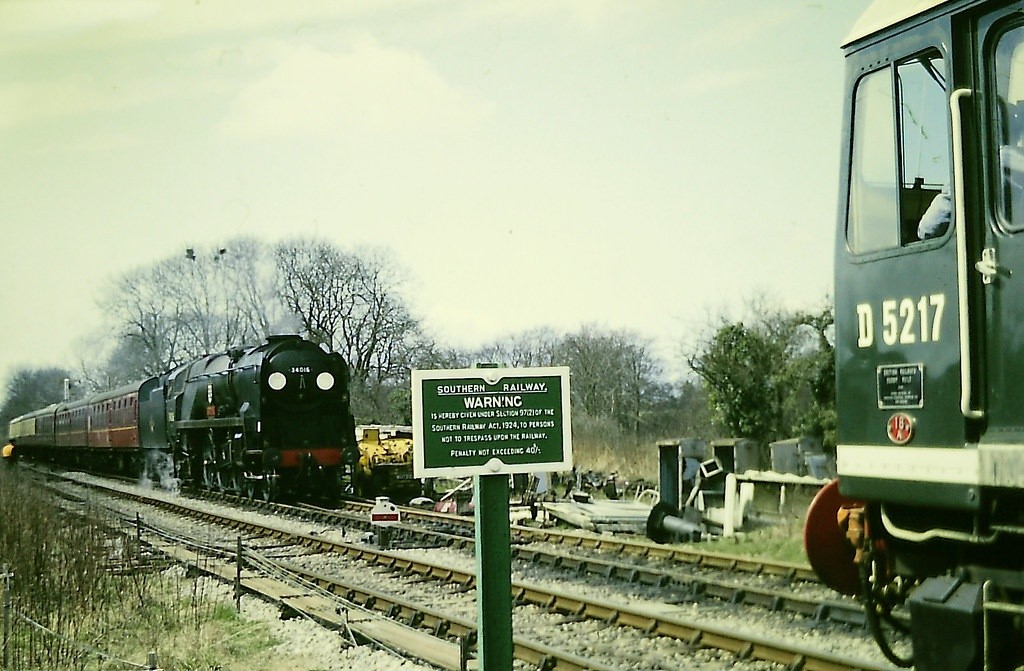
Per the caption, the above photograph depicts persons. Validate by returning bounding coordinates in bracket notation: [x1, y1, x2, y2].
[915, 175, 953, 239]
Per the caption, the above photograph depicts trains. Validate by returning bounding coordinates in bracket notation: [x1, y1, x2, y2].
[803, 1, 1024, 671]
[6, 336, 360, 503]
